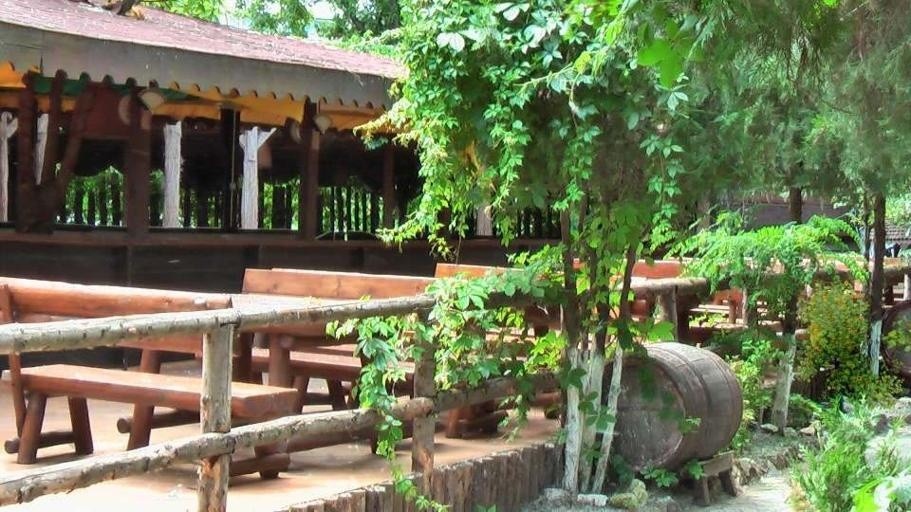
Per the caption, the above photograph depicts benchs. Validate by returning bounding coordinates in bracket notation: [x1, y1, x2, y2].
[0, 276, 298, 480]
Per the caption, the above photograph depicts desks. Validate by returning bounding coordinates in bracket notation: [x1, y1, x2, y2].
[126, 293, 380, 477]
